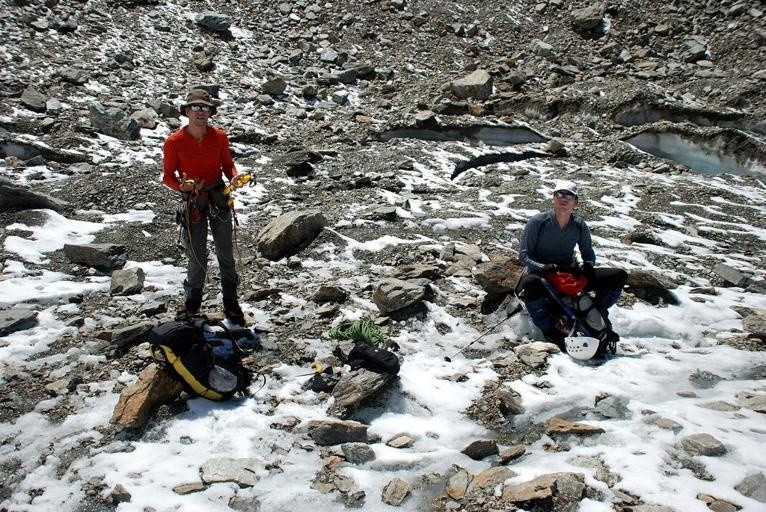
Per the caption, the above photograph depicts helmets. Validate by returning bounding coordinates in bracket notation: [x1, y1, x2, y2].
[564, 337, 599, 361]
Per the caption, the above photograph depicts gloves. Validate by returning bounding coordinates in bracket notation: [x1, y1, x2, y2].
[580, 262, 595, 271]
[543, 264, 558, 274]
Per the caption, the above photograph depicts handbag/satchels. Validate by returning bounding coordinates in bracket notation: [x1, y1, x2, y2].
[332, 344, 400, 374]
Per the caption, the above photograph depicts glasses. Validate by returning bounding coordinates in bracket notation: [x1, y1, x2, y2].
[554, 191, 575, 199]
[187, 105, 212, 112]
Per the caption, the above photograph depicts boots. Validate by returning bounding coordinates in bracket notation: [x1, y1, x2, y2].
[604, 311, 619, 343]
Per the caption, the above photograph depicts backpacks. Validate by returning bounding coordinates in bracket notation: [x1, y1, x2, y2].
[551, 271, 616, 355]
[149, 316, 255, 402]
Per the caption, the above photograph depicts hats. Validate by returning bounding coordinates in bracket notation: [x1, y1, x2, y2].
[180, 89, 217, 117]
[553, 181, 579, 197]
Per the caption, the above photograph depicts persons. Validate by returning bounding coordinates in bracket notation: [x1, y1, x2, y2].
[160, 88, 249, 324]
[517, 180, 629, 357]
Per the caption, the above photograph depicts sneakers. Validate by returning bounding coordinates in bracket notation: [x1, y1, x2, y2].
[223, 297, 244, 317]
[175, 297, 202, 319]
